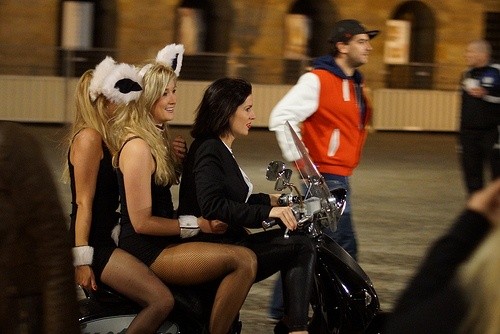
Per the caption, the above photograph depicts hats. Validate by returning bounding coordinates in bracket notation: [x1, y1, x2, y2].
[322, 19, 381, 58]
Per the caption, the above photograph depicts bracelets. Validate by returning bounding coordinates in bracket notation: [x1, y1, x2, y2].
[72, 244, 94, 266]
[179, 215, 200, 238]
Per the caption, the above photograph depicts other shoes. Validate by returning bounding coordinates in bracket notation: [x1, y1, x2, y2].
[274, 321, 290, 334]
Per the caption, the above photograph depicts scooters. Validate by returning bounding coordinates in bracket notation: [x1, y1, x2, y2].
[66, 121, 398, 334]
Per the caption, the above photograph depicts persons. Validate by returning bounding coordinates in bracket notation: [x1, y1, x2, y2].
[171, 78, 316, 334]
[68, 57, 176, 334]
[382, 179, 500, 334]
[456, 39, 500, 201]
[111, 43, 258, 334]
[267, 20, 382, 324]
[0, 121, 81, 334]
